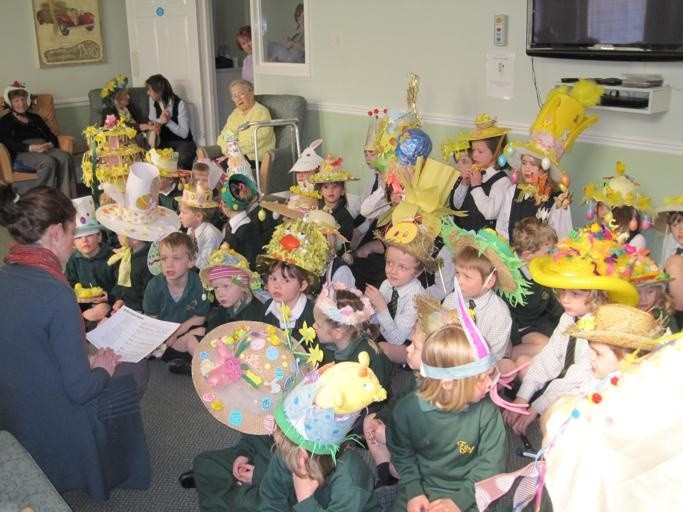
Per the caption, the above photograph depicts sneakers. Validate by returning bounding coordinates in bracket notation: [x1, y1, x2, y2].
[168, 357, 190, 375]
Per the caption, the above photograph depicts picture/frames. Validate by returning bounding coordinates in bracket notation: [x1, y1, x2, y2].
[32, 0, 107, 70]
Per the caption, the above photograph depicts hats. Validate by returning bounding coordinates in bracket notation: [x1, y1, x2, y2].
[652, 195, 683, 233]
[631, 252, 675, 288]
[415, 294, 461, 336]
[530, 226, 641, 305]
[222, 132, 359, 278]
[191, 321, 316, 436]
[314, 261, 375, 325]
[364, 74, 472, 275]
[273, 350, 388, 456]
[198, 251, 262, 291]
[69, 110, 222, 241]
[442, 223, 533, 308]
[4, 79, 32, 108]
[503, 79, 604, 189]
[467, 114, 511, 140]
[100, 75, 128, 104]
[563, 303, 665, 351]
[582, 161, 655, 230]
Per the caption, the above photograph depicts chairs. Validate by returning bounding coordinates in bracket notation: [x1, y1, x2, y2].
[154, 102, 196, 151]
[89, 86, 151, 149]
[202, 94, 307, 191]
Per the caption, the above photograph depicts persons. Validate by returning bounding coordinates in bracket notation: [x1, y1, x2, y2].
[282, 3, 304, 63]
[69, 73, 683, 511]
[236, 25, 295, 88]
[0, 186, 150, 502]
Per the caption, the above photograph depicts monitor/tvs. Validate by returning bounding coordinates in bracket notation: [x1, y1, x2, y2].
[525, 0, 683, 61]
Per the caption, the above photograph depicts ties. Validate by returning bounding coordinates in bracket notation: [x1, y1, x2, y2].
[562, 320, 581, 376]
[467, 299, 476, 325]
[480, 170, 486, 175]
[381, 289, 398, 342]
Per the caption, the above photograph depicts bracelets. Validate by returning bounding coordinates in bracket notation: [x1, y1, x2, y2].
[28, 144, 32, 152]
